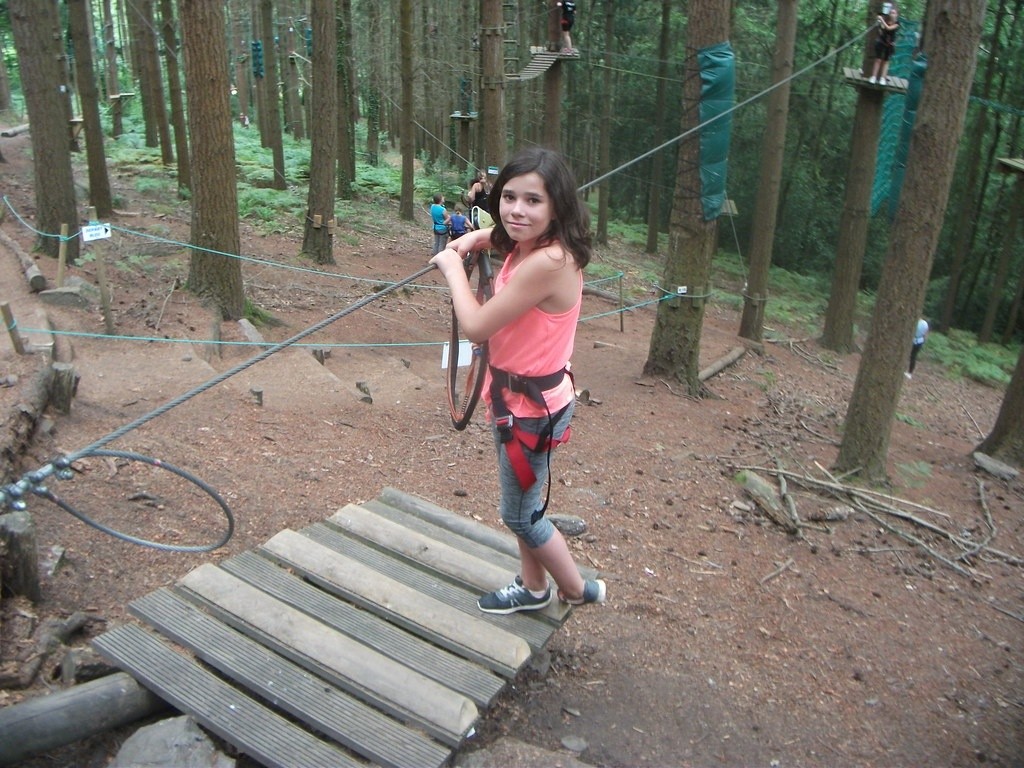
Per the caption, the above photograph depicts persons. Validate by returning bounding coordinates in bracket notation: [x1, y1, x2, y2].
[430, 193, 451, 256]
[869, 8, 900, 86]
[557, 0, 577, 54]
[903, 317, 929, 379]
[443, 203, 475, 240]
[468, 171, 493, 217]
[461, 179, 480, 224]
[428, 149, 606, 615]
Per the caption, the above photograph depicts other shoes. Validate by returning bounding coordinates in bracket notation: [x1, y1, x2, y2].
[904, 372, 912, 378]
[879, 77, 886, 85]
[869, 75, 876, 84]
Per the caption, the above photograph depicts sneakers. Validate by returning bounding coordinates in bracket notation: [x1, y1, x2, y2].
[557, 579, 606, 607]
[476, 575, 551, 615]
